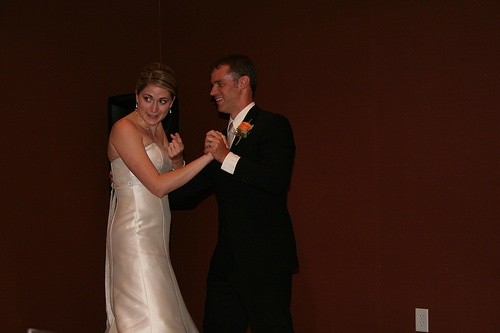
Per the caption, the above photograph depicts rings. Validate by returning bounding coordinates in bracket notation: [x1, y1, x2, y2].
[208, 141, 213, 148]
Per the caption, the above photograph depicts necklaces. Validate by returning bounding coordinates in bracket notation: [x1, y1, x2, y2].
[134, 110, 161, 142]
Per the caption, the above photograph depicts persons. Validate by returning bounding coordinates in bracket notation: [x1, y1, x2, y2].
[163, 52, 304, 333]
[97, 61, 230, 333]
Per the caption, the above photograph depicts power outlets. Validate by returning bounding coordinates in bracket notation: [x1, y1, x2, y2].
[415, 308, 429, 333]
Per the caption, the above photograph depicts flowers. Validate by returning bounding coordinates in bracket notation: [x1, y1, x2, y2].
[233, 121, 254, 145]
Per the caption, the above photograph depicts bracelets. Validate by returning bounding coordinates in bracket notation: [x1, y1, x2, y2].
[170, 159, 186, 172]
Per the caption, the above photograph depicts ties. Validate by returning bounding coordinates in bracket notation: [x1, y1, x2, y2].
[227, 122, 236, 150]
